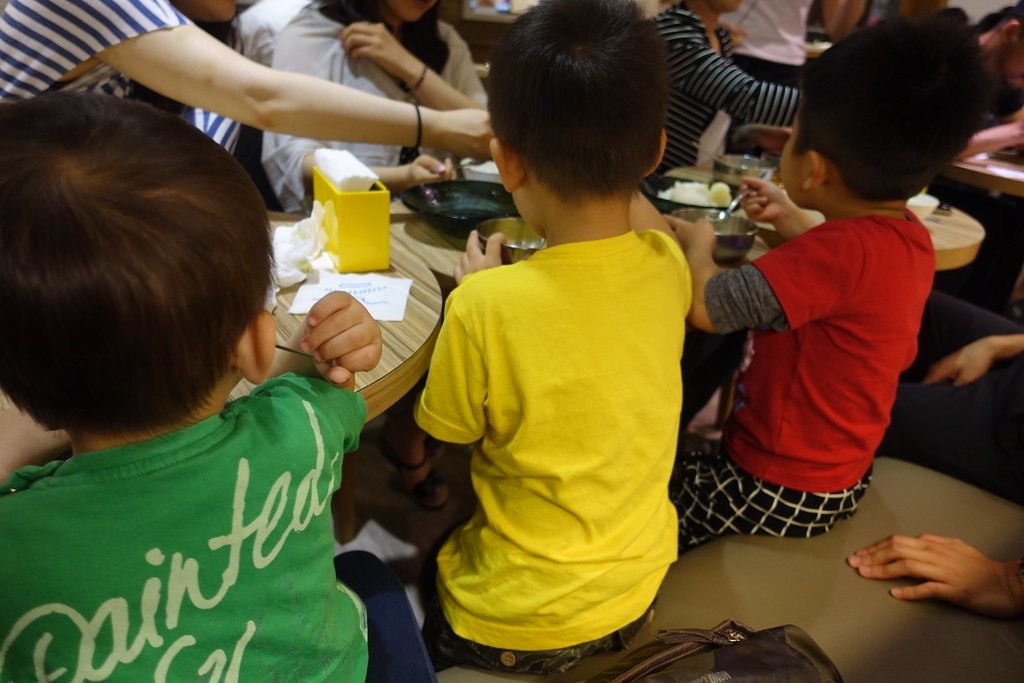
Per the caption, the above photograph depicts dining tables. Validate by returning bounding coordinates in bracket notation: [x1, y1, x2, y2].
[262, 209, 443, 427]
[389, 197, 469, 287]
[666, 165, 986, 271]
[939, 155, 1024, 196]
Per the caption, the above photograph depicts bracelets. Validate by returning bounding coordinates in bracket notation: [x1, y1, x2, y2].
[413, 103, 423, 149]
[407, 63, 428, 91]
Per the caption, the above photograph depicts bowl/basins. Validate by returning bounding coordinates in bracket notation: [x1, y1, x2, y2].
[475, 217, 546, 267]
[906, 194, 939, 223]
[673, 206, 756, 265]
[715, 153, 776, 190]
[642, 174, 740, 214]
[402, 180, 521, 238]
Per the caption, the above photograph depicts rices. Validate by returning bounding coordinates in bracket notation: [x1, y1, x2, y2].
[655, 180, 715, 208]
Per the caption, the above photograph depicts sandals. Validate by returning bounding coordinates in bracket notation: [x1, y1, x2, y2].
[377, 427, 453, 513]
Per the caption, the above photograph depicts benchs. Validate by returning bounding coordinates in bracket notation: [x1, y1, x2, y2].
[433, 456, 1024, 683]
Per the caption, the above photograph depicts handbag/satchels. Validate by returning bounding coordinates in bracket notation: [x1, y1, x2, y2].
[576, 620, 842, 683]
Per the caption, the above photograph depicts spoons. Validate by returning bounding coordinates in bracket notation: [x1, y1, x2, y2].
[705, 169, 773, 223]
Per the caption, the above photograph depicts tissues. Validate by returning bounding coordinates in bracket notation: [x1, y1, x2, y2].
[309, 146, 395, 275]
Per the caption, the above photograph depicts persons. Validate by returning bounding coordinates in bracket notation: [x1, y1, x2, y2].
[715, 0, 949, 90]
[415, 1, 693, 677]
[0, 0, 495, 161]
[847, 289, 1024, 621]
[260, 1, 490, 216]
[0, 92, 383, 682]
[928, 0, 1024, 313]
[636, 0, 806, 436]
[667, 13, 994, 557]
